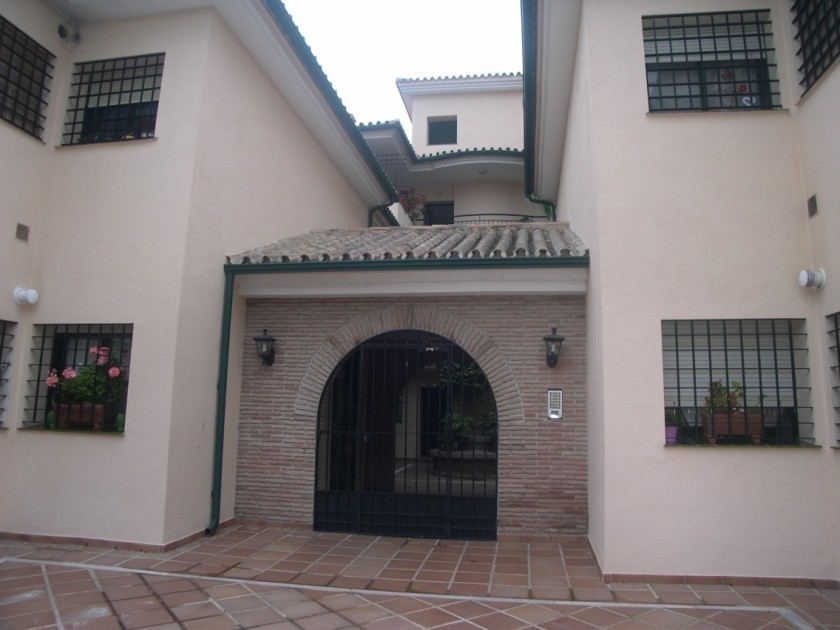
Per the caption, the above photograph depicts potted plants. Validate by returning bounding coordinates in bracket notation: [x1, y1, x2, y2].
[664, 408, 686, 445]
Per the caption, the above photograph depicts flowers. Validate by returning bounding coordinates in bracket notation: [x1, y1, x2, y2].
[44, 344, 129, 406]
[396, 180, 429, 225]
[702, 375, 768, 415]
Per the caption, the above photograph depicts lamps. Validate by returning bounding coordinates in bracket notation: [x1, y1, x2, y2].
[251, 327, 279, 368]
[541, 326, 566, 369]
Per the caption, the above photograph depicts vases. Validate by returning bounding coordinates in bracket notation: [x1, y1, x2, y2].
[701, 410, 765, 446]
[53, 400, 115, 433]
